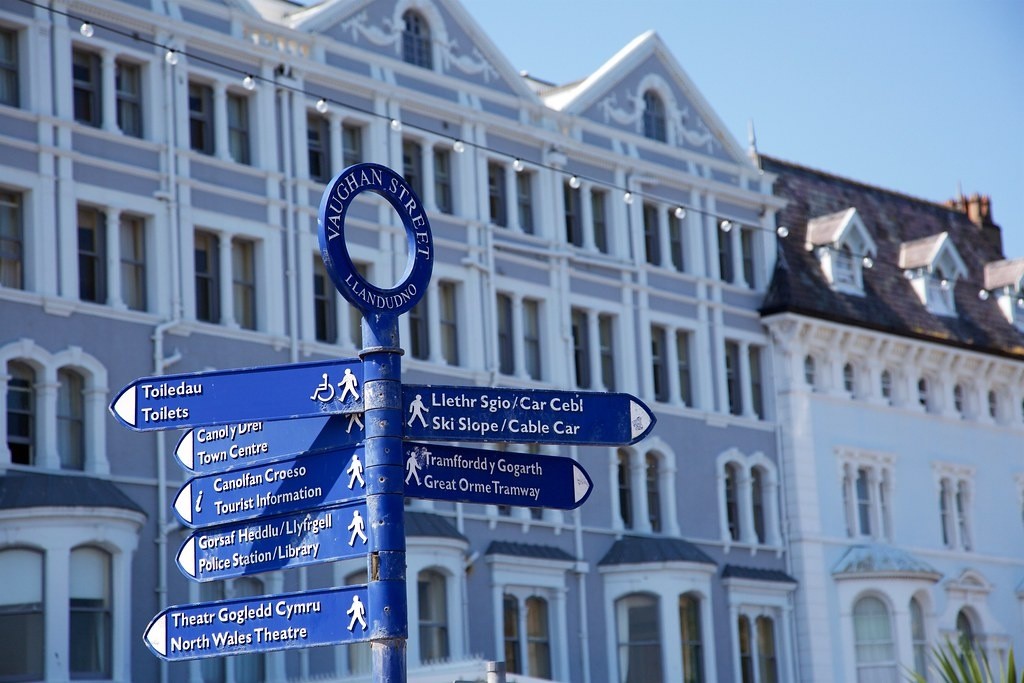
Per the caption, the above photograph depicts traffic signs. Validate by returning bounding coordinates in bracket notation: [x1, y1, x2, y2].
[174, 498, 368, 584]
[402, 439, 595, 510]
[141, 581, 369, 664]
[172, 411, 365, 477]
[106, 360, 365, 433]
[172, 446, 366, 531]
[402, 382, 657, 447]
